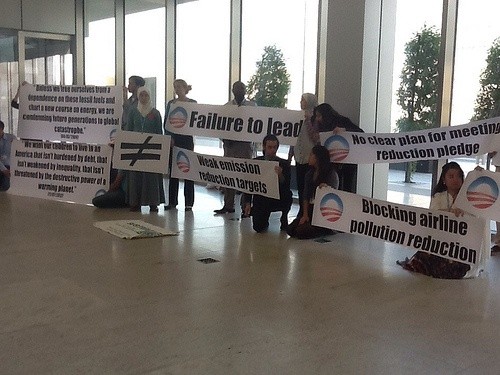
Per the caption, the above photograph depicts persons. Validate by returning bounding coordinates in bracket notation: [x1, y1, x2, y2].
[91, 75, 365, 240]
[0, 81, 43, 192]
[396, 149, 500, 280]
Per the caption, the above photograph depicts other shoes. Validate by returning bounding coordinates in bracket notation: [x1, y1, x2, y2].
[491, 245, 500, 252]
[280, 216, 288, 230]
[164, 204, 176, 209]
[150, 206, 158, 212]
[130, 205, 141, 211]
[214, 208, 235, 214]
[185, 204, 191, 211]
